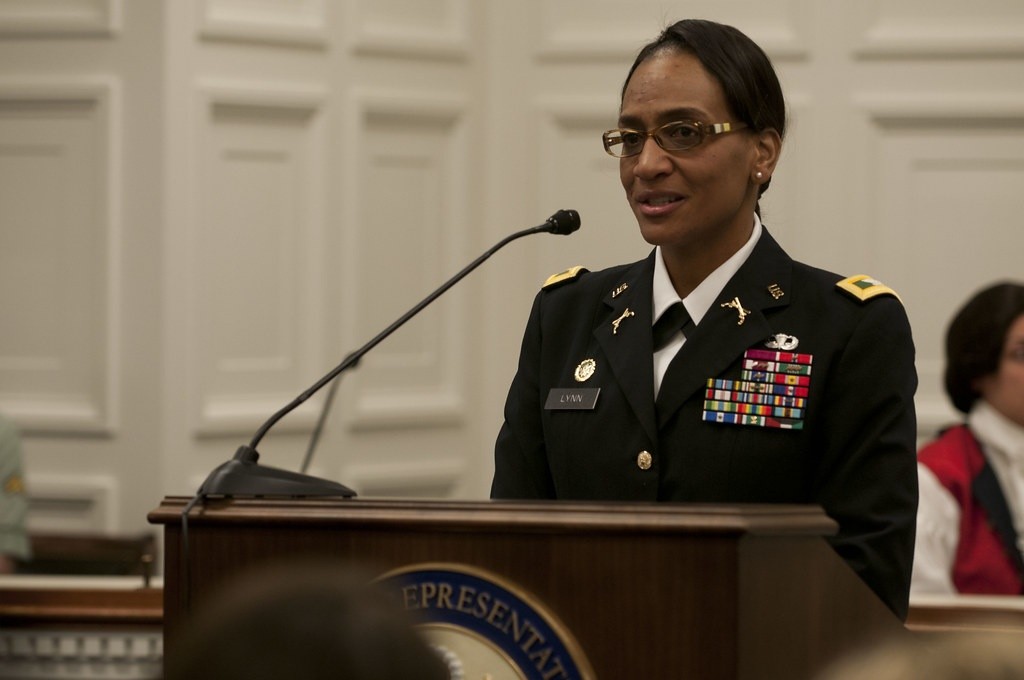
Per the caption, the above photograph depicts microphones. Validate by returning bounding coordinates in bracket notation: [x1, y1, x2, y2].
[197, 210, 581, 498]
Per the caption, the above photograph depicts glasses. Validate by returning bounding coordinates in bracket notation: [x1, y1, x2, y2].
[602, 119, 749, 158]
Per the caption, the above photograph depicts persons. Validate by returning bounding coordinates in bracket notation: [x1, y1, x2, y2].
[907, 281, 1024, 597]
[487, 17, 918, 621]
